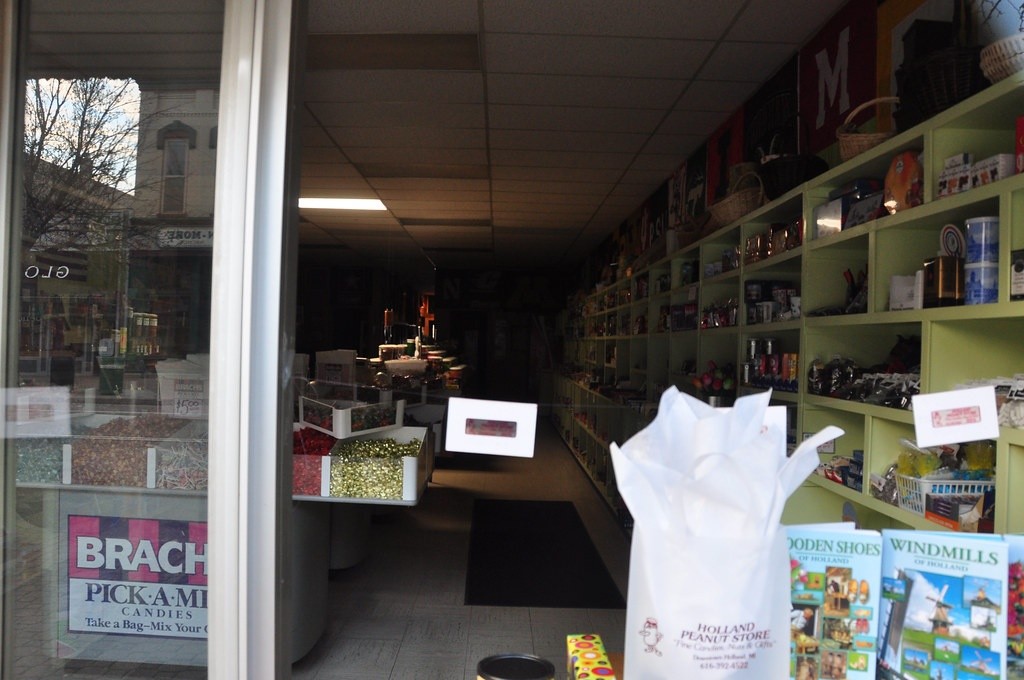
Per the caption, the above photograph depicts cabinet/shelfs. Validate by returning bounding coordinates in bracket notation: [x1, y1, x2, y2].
[544, 66, 1024, 545]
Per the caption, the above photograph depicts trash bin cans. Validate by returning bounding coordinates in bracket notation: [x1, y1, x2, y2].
[477, 653, 554, 680]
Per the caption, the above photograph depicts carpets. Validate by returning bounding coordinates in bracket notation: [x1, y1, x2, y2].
[461, 497, 628, 610]
